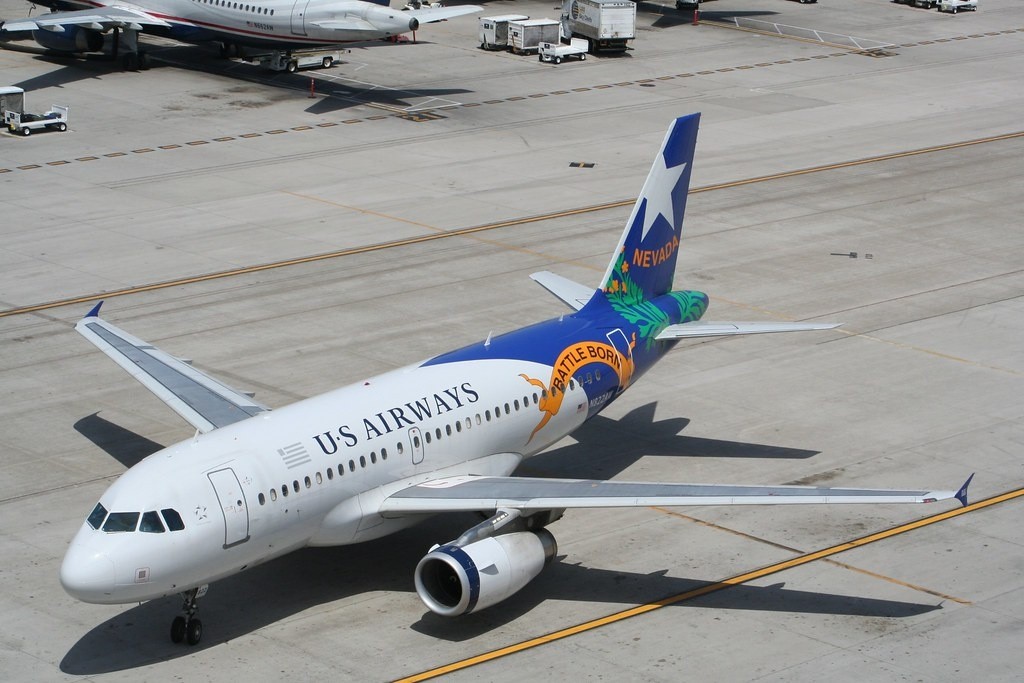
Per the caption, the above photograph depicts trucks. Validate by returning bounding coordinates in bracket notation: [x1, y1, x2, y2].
[562, 1, 635, 56]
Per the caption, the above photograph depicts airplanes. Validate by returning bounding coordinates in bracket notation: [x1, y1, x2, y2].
[0, 1, 484, 75]
[59, 110, 975, 647]
